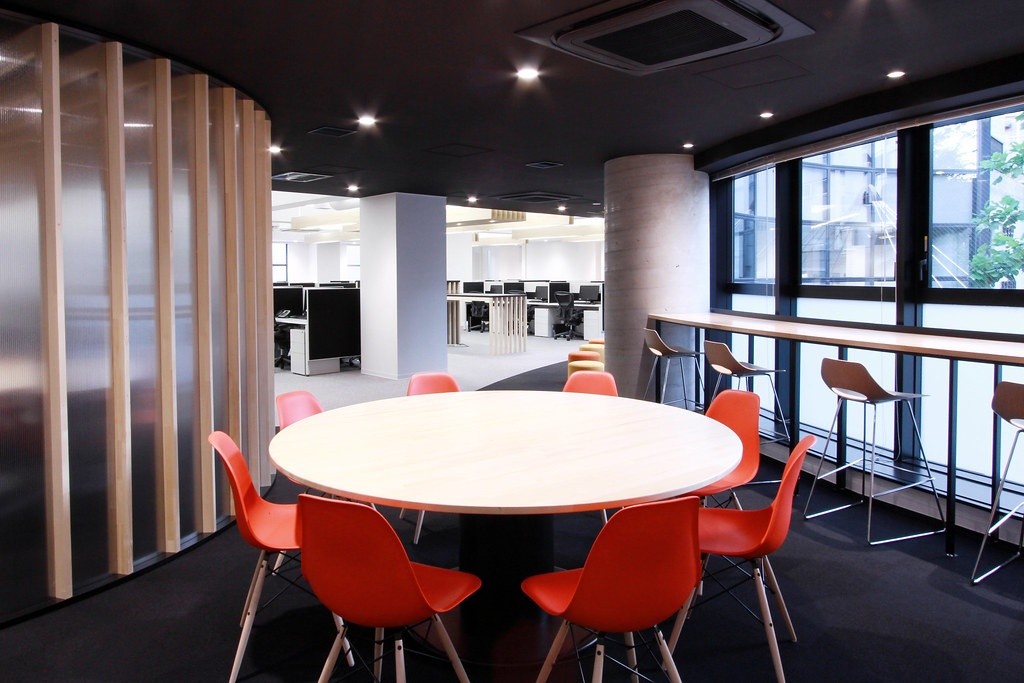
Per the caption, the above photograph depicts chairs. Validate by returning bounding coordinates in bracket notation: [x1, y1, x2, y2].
[273, 323, 294, 369]
[507, 289, 535, 336]
[208, 372, 816, 683]
[552, 290, 584, 341]
[468, 291, 490, 334]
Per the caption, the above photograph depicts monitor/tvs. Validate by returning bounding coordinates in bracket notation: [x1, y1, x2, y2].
[579, 286, 599, 304]
[491, 285, 503, 294]
[535, 286, 548, 301]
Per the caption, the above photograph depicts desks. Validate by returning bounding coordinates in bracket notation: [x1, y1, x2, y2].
[268, 390, 743, 683]
[650, 312, 1024, 367]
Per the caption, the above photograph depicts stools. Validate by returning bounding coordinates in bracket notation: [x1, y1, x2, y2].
[567, 339, 605, 380]
[642, 328, 704, 412]
[802, 358, 946, 545]
[967, 381, 1024, 585]
[704, 339, 790, 445]
